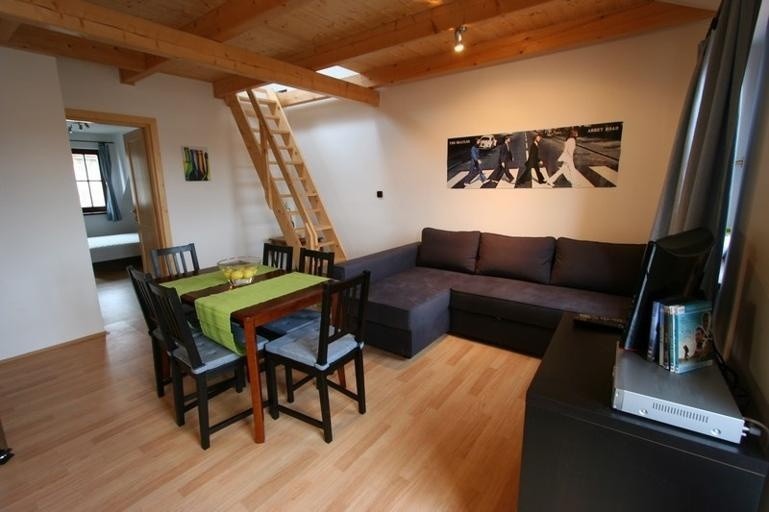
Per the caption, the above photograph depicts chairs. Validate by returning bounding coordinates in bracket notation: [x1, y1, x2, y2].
[263, 242, 293, 273]
[126, 264, 246, 398]
[150, 243, 200, 285]
[257, 247, 335, 344]
[146, 281, 270, 450]
[263, 270, 371, 444]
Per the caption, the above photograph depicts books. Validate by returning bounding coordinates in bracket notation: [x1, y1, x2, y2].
[647, 295, 716, 374]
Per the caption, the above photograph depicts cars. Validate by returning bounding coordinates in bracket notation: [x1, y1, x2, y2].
[476, 132, 513, 150]
[535, 129, 563, 138]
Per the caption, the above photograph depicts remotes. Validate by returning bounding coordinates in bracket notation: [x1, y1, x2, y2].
[573, 313, 624, 330]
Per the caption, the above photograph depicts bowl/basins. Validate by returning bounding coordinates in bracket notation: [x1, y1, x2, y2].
[218, 255, 262, 288]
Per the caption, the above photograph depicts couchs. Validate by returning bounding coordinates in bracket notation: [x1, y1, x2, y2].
[336, 240, 647, 359]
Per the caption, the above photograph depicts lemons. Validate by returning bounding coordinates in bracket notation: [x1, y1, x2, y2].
[222, 265, 258, 280]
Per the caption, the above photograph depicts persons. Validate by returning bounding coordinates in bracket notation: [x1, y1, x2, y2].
[516, 135, 547, 185]
[493, 135, 517, 187]
[463, 138, 490, 186]
[547, 131, 579, 187]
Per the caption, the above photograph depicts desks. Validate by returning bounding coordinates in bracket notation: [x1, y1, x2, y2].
[518, 311, 768, 511]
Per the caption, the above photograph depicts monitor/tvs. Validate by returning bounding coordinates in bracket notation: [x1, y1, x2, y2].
[619, 224, 722, 351]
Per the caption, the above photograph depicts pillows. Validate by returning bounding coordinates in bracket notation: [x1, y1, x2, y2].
[417, 227, 647, 298]
